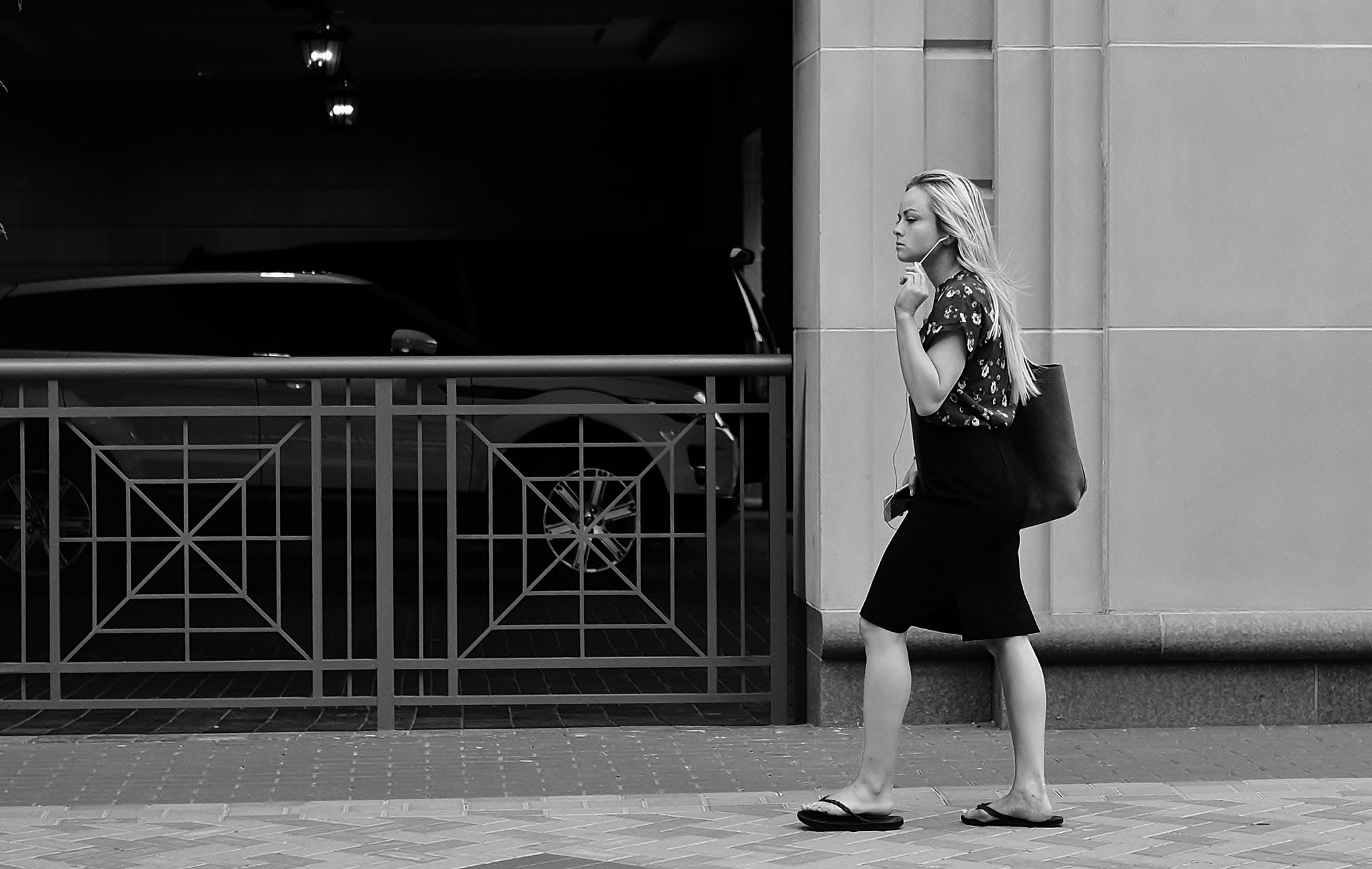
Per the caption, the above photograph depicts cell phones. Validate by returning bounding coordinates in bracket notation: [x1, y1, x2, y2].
[883, 481, 913, 522]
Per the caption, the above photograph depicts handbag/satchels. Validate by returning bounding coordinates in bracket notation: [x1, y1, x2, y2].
[1007, 364, 1088, 529]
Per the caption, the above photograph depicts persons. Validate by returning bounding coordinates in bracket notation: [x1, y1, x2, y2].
[796, 168, 1064, 833]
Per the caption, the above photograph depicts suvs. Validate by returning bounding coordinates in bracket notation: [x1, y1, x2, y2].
[0, 271, 741, 600]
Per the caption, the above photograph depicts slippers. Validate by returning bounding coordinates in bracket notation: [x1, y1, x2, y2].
[797, 795, 904, 831]
[960, 802, 1063, 828]
